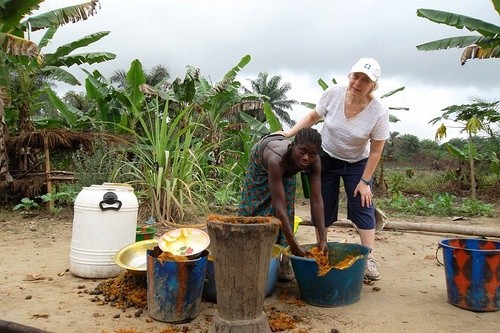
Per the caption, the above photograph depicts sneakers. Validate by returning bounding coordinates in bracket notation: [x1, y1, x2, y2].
[364, 257, 382, 280]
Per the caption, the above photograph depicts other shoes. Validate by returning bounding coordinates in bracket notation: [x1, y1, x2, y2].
[278, 259, 295, 279]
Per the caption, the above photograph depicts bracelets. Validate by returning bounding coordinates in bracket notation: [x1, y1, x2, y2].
[360, 178, 370, 185]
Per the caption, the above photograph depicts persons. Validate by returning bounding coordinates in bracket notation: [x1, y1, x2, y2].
[270, 57, 389, 280]
[237, 127, 328, 281]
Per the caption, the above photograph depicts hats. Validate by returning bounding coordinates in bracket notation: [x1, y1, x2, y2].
[351, 57, 381, 81]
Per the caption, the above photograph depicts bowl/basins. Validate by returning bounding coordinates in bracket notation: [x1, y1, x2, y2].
[136, 227, 156, 242]
[200, 243, 284, 303]
[293, 215, 303, 235]
[114, 239, 160, 277]
[159, 227, 210, 258]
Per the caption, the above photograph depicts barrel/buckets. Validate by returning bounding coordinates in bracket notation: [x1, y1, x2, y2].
[284, 242, 376, 309]
[436, 238, 500, 312]
[69, 186, 139, 281]
[146, 245, 209, 324]
[353, 207, 387, 236]
